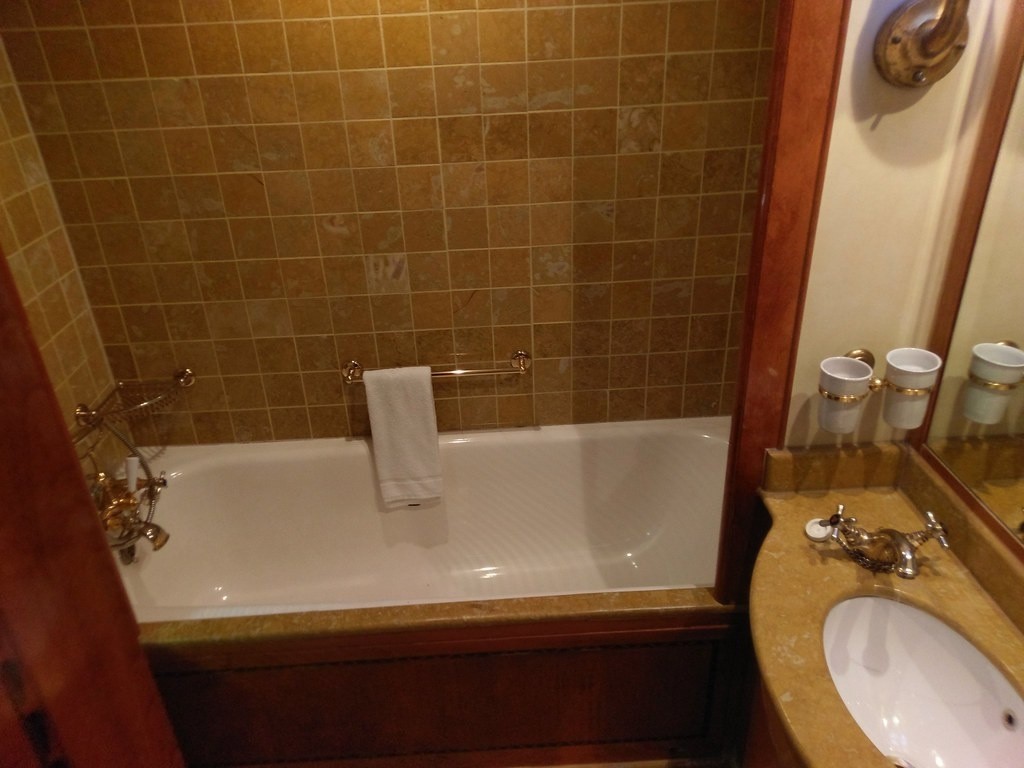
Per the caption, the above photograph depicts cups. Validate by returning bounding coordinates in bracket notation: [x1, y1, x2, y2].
[821, 356, 873, 435]
[885, 349, 941, 429]
[965, 343, 1024, 424]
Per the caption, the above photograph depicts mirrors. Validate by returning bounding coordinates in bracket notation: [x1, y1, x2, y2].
[910, 0, 1024, 562]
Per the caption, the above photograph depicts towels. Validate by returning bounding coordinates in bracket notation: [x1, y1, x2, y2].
[361, 365, 446, 511]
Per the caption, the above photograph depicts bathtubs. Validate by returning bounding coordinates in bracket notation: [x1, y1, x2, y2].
[115, 414, 732, 766]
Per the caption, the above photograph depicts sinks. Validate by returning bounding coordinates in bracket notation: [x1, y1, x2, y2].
[821, 587, 1023, 768]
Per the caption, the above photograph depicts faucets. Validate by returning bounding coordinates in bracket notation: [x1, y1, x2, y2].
[819, 501, 950, 582]
[121, 515, 171, 553]
